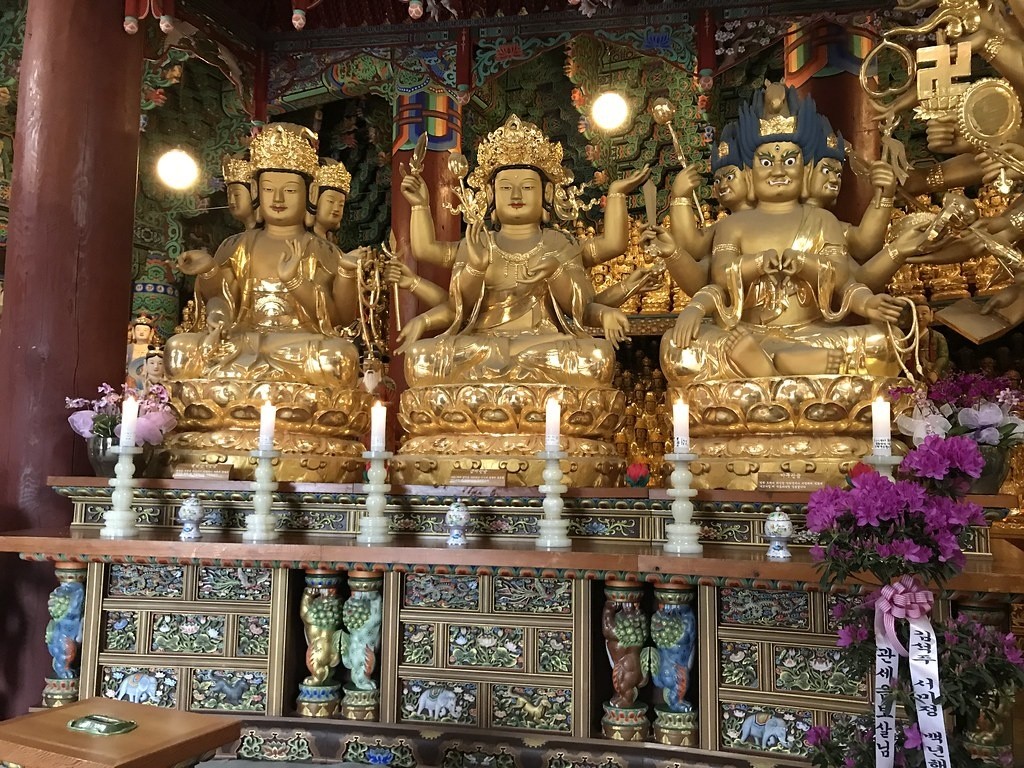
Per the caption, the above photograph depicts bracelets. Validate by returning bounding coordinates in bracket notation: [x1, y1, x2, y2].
[871, 196, 895, 207]
[888, 242, 906, 264]
[928, 165, 945, 191]
[796, 251, 805, 273]
[338, 266, 356, 278]
[755, 252, 765, 276]
[607, 192, 626, 199]
[980, 35, 1005, 62]
[409, 276, 422, 292]
[620, 281, 632, 298]
[199, 263, 219, 279]
[1010, 211, 1024, 233]
[687, 301, 707, 317]
[422, 311, 431, 330]
[412, 205, 430, 209]
[670, 198, 693, 205]
[285, 272, 304, 291]
[597, 306, 618, 324]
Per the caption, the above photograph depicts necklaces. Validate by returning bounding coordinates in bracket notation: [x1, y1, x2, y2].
[488, 231, 544, 278]
[664, 246, 683, 262]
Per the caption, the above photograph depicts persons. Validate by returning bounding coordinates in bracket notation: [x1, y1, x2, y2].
[956, 335, 1024, 518]
[639, 86, 953, 382]
[385, 116, 667, 388]
[871, 1, 1023, 328]
[904, 301, 949, 384]
[613, 338, 672, 489]
[358, 352, 397, 469]
[125, 315, 169, 402]
[163, 128, 378, 384]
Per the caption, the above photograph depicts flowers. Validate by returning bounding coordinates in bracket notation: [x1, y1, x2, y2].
[804, 357, 1024, 768]
[64, 383, 177, 446]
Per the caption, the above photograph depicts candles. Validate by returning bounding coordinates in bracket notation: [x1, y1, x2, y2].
[545, 398, 560, 446]
[672, 398, 691, 453]
[370, 400, 386, 447]
[120, 397, 138, 447]
[259, 400, 276, 443]
[872, 396, 892, 456]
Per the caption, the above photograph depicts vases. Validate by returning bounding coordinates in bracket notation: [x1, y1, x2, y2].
[86, 438, 150, 479]
[963, 445, 1011, 495]
